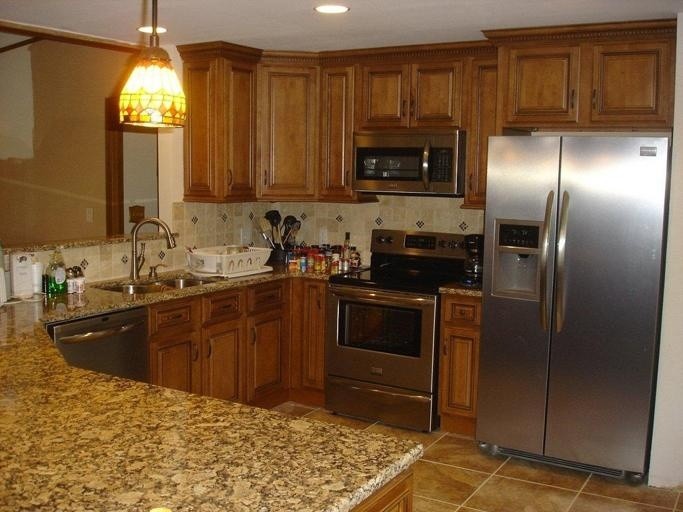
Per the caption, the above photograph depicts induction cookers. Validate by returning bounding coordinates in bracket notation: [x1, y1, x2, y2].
[327, 251, 464, 297]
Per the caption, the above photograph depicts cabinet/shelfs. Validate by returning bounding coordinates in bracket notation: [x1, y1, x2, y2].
[285, 275, 326, 414]
[353, 43, 460, 129]
[316, 50, 375, 205]
[256, 47, 315, 199]
[146, 282, 240, 406]
[434, 285, 483, 436]
[177, 41, 256, 204]
[464, 41, 496, 210]
[479, 19, 677, 131]
[240, 280, 286, 408]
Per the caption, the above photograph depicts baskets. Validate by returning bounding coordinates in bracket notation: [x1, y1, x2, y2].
[185, 245, 273, 275]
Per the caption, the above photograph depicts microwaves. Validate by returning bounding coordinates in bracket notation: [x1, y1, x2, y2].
[352, 130, 466, 200]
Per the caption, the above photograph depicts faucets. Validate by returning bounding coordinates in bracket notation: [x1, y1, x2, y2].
[129, 215, 177, 280]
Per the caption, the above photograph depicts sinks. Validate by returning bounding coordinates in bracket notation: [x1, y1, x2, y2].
[157, 275, 215, 290]
[88, 280, 173, 297]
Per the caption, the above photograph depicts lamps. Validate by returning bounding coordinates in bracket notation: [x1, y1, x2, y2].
[113, 0, 188, 130]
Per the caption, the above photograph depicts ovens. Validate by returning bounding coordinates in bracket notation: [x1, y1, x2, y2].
[324, 284, 436, 436]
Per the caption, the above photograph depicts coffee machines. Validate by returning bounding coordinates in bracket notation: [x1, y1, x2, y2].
[459, 234, 484, 289]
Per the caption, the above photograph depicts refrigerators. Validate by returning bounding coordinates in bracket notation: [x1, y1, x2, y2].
[473, 131, 672, 486]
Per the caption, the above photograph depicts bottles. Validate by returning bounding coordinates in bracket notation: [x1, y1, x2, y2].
[287, 244, 359, 274]
[44, 293, 85, 309]
[31, 246, 84, 293]
[343, 231, 351, 249]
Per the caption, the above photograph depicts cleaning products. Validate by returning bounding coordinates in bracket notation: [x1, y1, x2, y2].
[0, 246, 66, 306]
